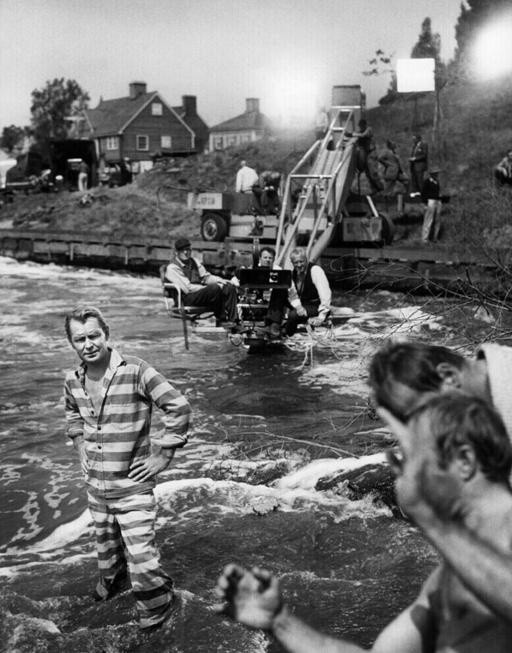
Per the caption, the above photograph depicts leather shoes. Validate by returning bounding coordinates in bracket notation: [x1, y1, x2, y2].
[233, 324, 251, 333]
[216, 319, 237, 328]
[257, 326, 270, 335]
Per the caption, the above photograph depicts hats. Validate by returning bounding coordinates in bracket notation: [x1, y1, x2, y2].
[429, 165, 441, 173]
[175, 238, 191, 248]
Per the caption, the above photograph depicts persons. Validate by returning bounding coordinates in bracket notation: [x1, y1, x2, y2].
[231, 248, 282, 287]
[213, 395, 512, 653]
[367, 337, 512, 439]
[254, 248, 332, 335]
[234, 119, 442, 243]
[496, 150, 512, 185]
[164, 238, 253, 334]
[62, 308, 192, 634]
[77, 161, 90, 190]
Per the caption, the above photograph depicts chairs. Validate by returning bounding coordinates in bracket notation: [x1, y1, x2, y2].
[296, 308, 343, 370]
[158, 264, 214, 350]
